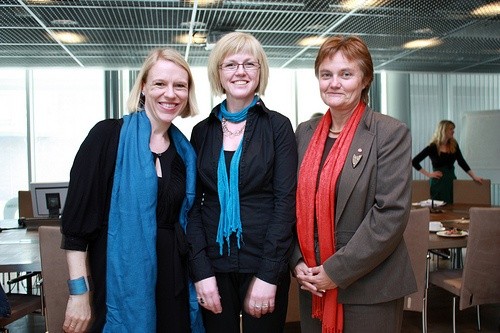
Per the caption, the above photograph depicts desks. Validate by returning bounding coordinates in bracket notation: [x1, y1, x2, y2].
[0, 228, 48, 272]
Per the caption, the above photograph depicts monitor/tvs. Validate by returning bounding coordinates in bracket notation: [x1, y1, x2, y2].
[30, 182, 69, 217]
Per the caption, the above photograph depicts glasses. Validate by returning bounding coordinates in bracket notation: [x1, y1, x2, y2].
[218, 61, 261, 70]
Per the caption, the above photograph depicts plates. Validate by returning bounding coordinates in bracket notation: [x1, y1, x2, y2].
[429, 227, 446, 232]
[436, 231, 467, 237]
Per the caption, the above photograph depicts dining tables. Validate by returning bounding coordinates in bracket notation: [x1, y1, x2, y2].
[411, 203, 500, 270]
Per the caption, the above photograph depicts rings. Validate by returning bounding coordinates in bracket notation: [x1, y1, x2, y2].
[262, 305, 268, 308]
[255, 305, 261, 308]
[200, 298, 206, 304]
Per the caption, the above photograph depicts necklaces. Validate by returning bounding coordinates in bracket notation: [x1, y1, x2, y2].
[329, 128, 343, 134]
[222, 117, 246, 136]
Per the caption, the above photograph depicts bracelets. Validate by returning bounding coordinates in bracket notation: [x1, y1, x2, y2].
[67, 276, 87, 295]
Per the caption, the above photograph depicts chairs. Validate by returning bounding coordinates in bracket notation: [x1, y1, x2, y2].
[429, 207, 500, 333]
[404, 208, 429, 333]
[0, 263, 42, 333]
[39, 226, 97, 333]
[453, 179, 491, 203]
[409, 180, 430, 204]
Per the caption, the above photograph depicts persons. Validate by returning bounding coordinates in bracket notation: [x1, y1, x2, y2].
[60, 47, 195, 333]
[186, 32, 297, 333]
[412, 119, 484, 204]
[289, 35, 412, 333]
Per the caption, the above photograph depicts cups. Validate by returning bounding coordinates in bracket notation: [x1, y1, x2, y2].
[429, 221, 443, 229]
